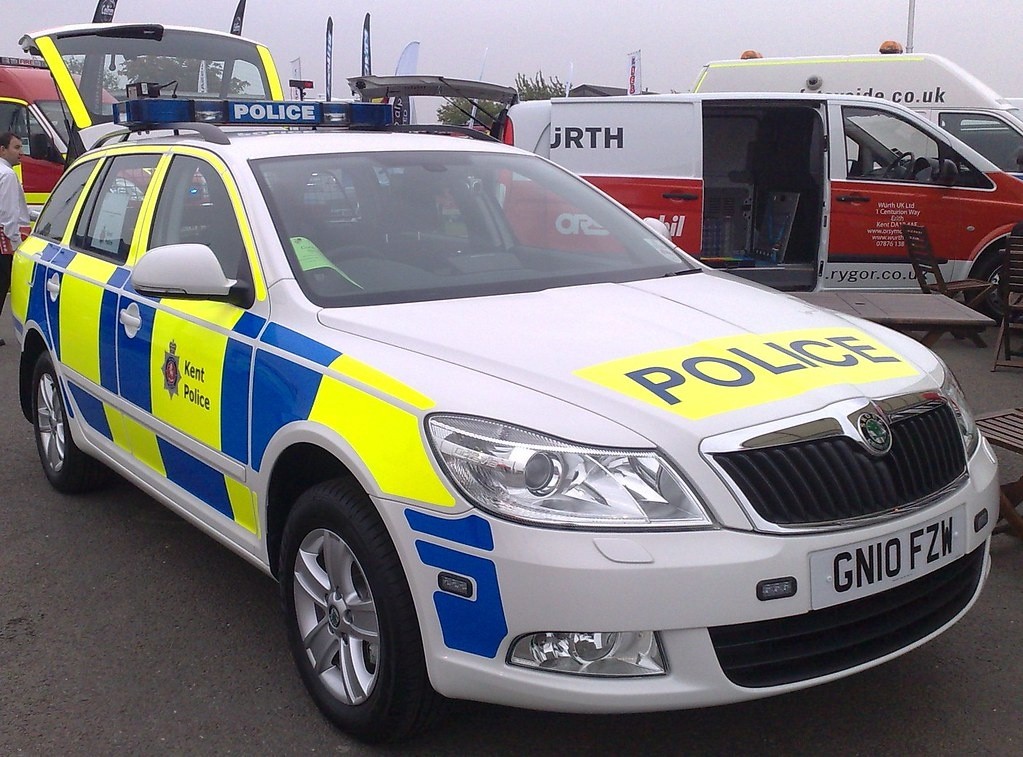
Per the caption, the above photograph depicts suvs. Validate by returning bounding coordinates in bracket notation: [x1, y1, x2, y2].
[12, 21, 1001, 750]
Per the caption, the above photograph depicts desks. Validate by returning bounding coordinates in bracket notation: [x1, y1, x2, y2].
[786, 291, 996, 349]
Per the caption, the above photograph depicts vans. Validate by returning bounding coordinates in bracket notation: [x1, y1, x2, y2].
[692, 41, 1022, 180]
[347, 75, 1023, 327]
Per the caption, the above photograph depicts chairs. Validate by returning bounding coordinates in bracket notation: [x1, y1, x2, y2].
[973, 406, 1023, 539]
[901, 223, 991, 349]
[990, 222, 1023, 373]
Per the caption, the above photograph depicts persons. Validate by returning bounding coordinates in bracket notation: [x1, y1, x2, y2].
[0, 131, 32, 346]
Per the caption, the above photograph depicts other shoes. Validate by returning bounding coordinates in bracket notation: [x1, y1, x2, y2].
[0, 338, 6, 346]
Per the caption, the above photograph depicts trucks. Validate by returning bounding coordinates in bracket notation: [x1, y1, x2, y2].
[0, 58, 212, 229]
